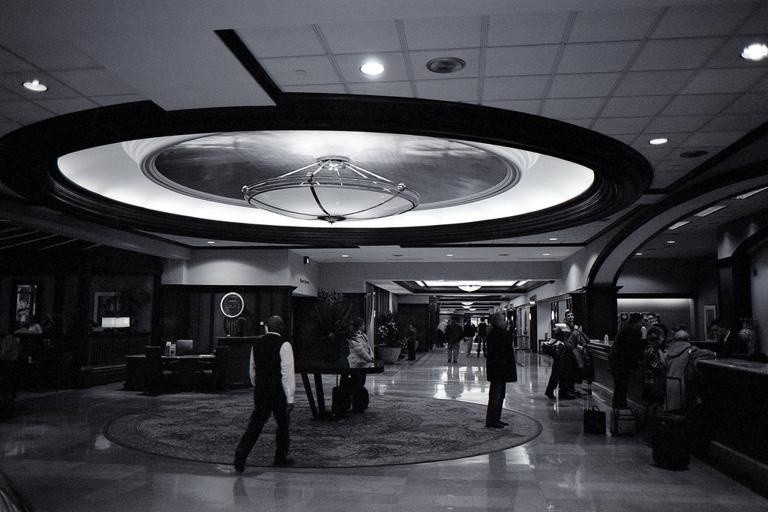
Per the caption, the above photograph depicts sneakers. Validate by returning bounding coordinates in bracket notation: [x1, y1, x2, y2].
[487, 421, 508, 428]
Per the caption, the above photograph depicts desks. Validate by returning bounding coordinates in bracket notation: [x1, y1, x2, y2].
[123, 354, 215, 390]
[294, 365, 385, 418]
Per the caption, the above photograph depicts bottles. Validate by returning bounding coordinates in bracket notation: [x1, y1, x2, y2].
[604, 334, 608, 345]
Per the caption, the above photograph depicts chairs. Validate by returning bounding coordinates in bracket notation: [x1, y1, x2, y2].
[142, 343, 182, 390]
[175, 339, 194, 354]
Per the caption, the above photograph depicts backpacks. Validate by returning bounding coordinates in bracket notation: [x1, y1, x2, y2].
[360, 388, 369, 408]
[610, 407, 639, 437]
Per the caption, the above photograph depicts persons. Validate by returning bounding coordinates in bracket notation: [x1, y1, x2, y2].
[486, 312, 517, 428]
[711, 318, 749, 359]
[544, 311, 578, 400]
[233, 315, 297, 474]
[406, 324, 417, 361]
[608, 312, 715, 415]
[436, 319, 448, 347]
[346, 318, 375, 414]
[463, 317, 475, 357]
[445, 316, 462, 363]
[12, 316, 42, 333]
[476, 317, 487, 358]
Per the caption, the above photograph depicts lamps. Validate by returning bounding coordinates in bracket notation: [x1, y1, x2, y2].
[239, 156, 421, 227]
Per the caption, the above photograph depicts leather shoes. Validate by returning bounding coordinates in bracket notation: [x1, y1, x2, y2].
[544, 388, 581, 400]
[274, 456, 288, 465]
[235, 449, 247, 473]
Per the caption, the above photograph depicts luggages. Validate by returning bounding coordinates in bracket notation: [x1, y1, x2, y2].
[332, 387, 351, 413]
[652, 375, 692, 470]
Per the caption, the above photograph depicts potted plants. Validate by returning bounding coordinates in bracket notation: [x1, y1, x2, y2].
[378, 330, 407, 363]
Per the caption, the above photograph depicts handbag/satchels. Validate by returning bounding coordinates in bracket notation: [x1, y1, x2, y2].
[542, 338, 565, 358]
[584, 405, 606, 435]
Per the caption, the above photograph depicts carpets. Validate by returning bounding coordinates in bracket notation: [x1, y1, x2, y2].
[102, 390, 543, 469]
